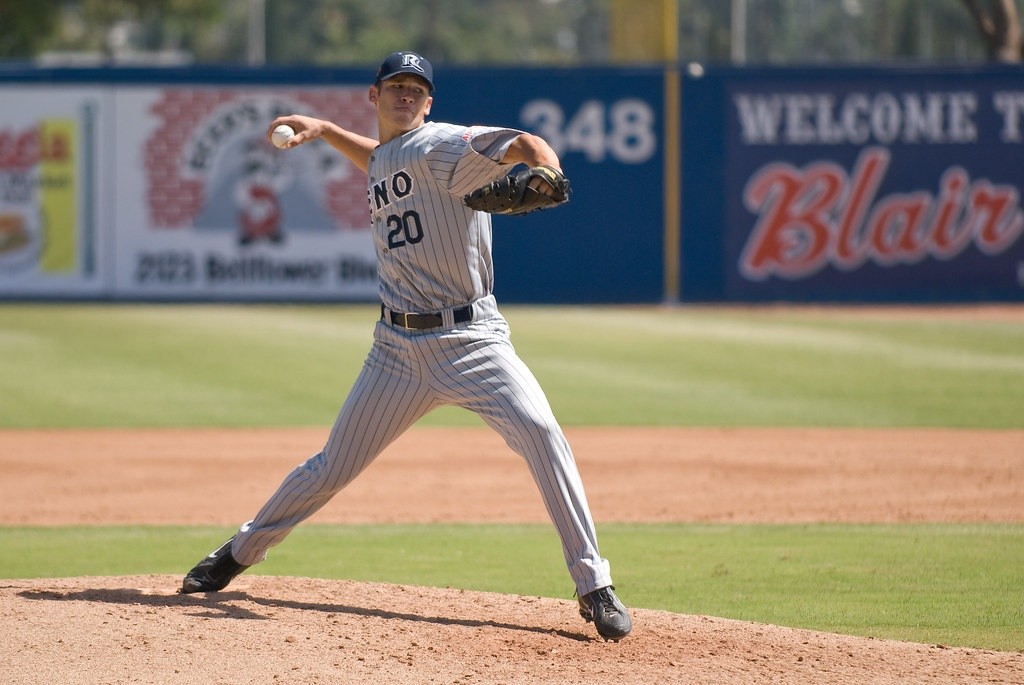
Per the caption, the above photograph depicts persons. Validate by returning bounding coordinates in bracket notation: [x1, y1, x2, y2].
[181, 50, 632, 642]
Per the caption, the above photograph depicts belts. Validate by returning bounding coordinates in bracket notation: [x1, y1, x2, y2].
[382, 304, 473, 330]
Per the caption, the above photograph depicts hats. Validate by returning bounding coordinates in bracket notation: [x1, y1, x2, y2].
[376, 51, 435, 93]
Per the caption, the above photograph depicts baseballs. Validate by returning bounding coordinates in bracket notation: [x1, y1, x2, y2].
[270, 125, 295, 150]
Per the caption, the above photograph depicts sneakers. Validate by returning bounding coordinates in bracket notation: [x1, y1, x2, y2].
[580, 585, 632, 642]
[183, 535, 251, 594]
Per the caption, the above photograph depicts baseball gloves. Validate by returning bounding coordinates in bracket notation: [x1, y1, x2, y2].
[462, 163, 573, 216]
